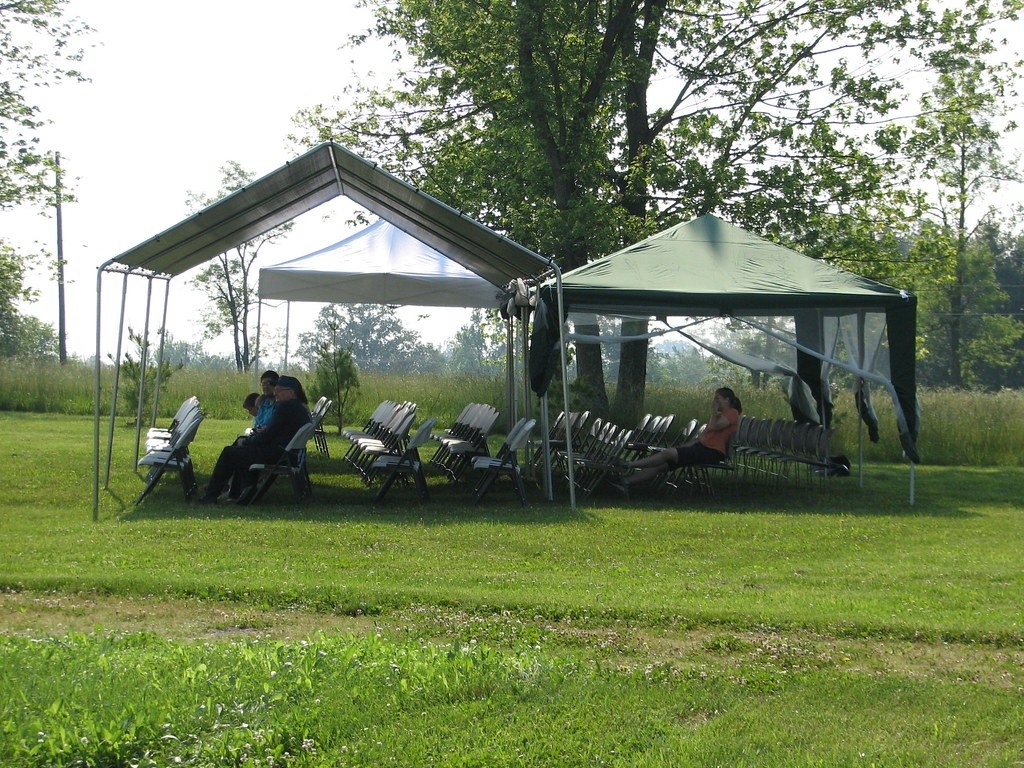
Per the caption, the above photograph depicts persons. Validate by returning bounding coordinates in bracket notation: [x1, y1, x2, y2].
[199, 370, 313, 507]
[610, 388, 742, 488]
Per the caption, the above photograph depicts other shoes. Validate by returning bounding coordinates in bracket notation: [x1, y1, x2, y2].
[604, 478, 626, 493]
[215, 490, 230, 499]
[223, 496, 241, 505]
[611, 456, 629, 471]
[199, 495, 217, 504]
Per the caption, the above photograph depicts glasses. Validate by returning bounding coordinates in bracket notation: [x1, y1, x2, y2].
[259, 381, 272, 386]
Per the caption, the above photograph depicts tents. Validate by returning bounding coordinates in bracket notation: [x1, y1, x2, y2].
[92, 141, 577, 528]
[507, 212, 918, 510]
[255, 219, 516, 464]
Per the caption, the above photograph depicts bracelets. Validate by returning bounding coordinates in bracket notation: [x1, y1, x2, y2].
[711, 412, 719, 416]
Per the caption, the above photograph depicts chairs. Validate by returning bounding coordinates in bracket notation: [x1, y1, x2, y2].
[133, 395, 207, 507]
[238, 396, 333, 509]
[342, 400, 836, 509]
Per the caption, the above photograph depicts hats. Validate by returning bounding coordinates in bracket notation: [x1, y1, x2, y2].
[276, 375, 298, 389]
